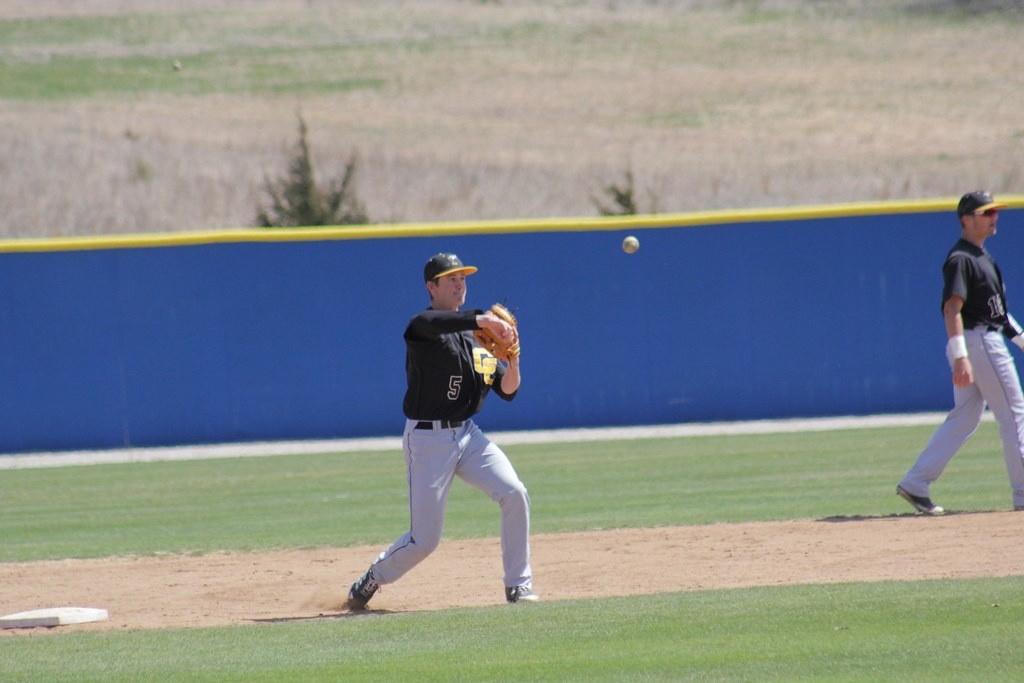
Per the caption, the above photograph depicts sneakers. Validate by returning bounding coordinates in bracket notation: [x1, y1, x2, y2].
[897, 486, 945, 516]
[507, 585, 539, 601]
[349, 569, 378, 612]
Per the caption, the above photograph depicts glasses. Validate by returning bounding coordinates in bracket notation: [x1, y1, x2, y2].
[976, 209, 998, 215]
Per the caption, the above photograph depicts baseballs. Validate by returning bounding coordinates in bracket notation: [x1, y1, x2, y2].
[623, 236, 639, 254]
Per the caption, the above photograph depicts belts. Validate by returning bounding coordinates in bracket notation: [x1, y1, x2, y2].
[414, 420, 466, 430]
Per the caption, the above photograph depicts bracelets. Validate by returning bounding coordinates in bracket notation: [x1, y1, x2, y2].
[949, 333, 968, 358]
[1011, 333, 1024, 351]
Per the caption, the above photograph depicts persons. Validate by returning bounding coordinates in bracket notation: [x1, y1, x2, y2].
[895, 188, 1024, 518]
[349, 253, 545, 612]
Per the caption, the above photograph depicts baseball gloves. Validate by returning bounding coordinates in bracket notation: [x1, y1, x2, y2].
[473, 303, 521, 362]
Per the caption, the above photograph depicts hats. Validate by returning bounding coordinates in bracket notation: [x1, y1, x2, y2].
[424, 252, 477, 281]
[957, 191, 1009, 216]
